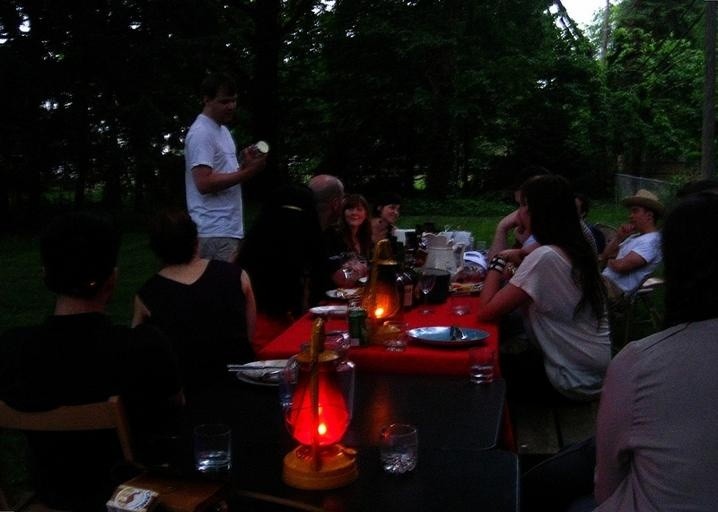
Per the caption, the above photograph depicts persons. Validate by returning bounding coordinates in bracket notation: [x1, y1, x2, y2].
[235, 180, 322, 326]
[585, 179, 717, 511]
[184, 74, 264, 263]
[131, 208, 255, 395]
[333, 196, 367, 257]
[308, 173, 345, 273]
[370, 193, 400, 245]
[0, 205, 189, 512]
[475, 176, 663, 415]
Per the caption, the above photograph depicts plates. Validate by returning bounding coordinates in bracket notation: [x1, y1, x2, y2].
[310, 305, 349, 317]
[448, 285, 484, 293]
[326, 288, 356, 299]
[406, 325, 490, 347]
[241, 358, 297, 384]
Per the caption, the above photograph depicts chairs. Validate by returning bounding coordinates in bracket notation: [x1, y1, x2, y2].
[0, 395, 231, 512]
[626, 267, 666, 336]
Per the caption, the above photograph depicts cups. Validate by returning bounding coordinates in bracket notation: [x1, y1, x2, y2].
[378, 424, 419, 475]
[191, 422, 231, 472]
[467, 345, 497, 384]
[378, 318, 406, 353]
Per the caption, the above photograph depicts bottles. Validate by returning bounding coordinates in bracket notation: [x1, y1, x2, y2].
[380, 235, 420, 311]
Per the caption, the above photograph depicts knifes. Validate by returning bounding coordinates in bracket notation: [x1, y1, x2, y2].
[220, 365, 287, 371]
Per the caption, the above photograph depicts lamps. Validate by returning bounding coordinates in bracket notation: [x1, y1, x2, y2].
[276, 318, 361, 489]
[365, 238, 410, 350]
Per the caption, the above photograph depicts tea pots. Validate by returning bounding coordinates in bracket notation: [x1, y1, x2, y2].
[418, 232, 466, 284]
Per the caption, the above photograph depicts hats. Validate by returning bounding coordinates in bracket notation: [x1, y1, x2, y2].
[622, 190, 664, 214]
